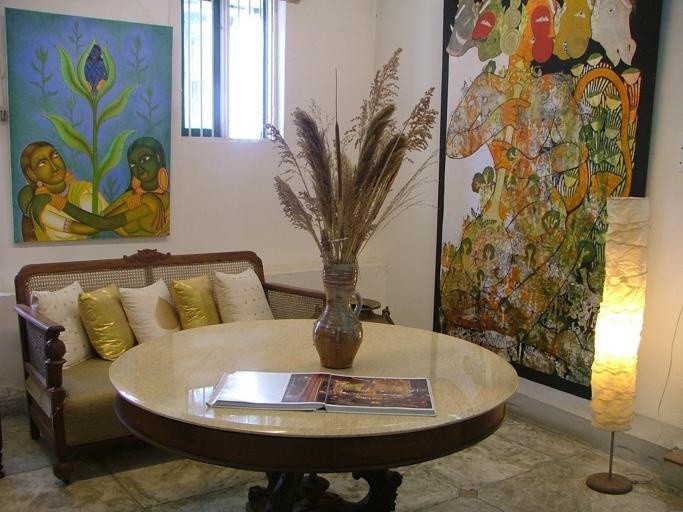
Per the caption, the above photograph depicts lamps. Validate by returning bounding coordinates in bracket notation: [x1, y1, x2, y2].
[585, 196, 651, 495]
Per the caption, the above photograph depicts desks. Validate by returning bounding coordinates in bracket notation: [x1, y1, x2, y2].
[108, 318, 519, 512]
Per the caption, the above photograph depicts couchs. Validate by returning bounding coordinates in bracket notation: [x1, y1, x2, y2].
[13, 248, 395, 486]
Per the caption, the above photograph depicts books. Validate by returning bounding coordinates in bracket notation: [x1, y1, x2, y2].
[207, 368, 437, 417]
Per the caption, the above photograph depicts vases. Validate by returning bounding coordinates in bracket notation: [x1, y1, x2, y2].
[312, 262, 363, 369]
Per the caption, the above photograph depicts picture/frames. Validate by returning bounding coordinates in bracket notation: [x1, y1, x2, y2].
[432, 0, 664, 400]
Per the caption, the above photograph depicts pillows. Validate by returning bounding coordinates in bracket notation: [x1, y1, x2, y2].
[170, 274, 222, 331]
[78, 282, 138, 361]
[213, 266, 275, 323]
[29, 280, 98, 371]
[117, 278, 182, 345]
